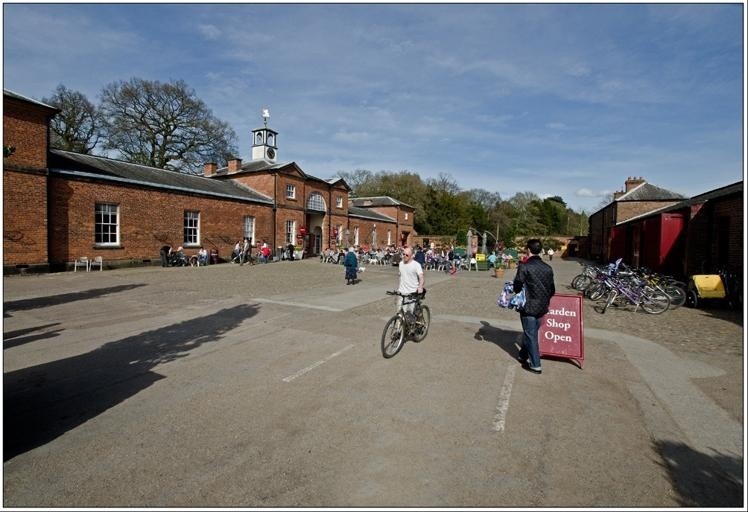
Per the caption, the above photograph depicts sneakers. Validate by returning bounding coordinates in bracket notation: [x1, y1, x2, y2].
[529, 365, 542, 374]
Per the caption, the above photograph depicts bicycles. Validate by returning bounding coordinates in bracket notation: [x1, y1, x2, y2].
[377, 288, 431, 360]
[568, 257, 687, 317]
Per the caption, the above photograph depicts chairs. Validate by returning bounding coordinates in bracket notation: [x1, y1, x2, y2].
[73, 255, 102, 273]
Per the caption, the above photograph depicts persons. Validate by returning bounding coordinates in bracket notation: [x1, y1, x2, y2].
[343, 246, 357, 285]
[501, 248, 513, 269]
[520, 243, 530, 253]
[538, 248, 545, 260]
[231, 239, 271, 266]
[471, 241, 477, 258]
[390, 247, 424, 341]
[488, 251, 498, 268]
[160, 244, 184, 267]
[518, 250, 529, 266]
[190, 247, 218, 267]
[320, 242, 468, 273]
[511, 239, 554, 373]
[547, 248, 553, 260]
[285, 243, 294, 261]
[496, 243, 505, 253]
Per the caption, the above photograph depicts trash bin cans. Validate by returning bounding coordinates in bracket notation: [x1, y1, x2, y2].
[210, 249, 218, 264]
[276, 246, 284, 260]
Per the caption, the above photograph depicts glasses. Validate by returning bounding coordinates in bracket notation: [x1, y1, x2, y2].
[403, 253, 409, 257]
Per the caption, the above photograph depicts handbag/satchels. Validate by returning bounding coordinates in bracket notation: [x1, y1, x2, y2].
[498, 281, 527, 312]
